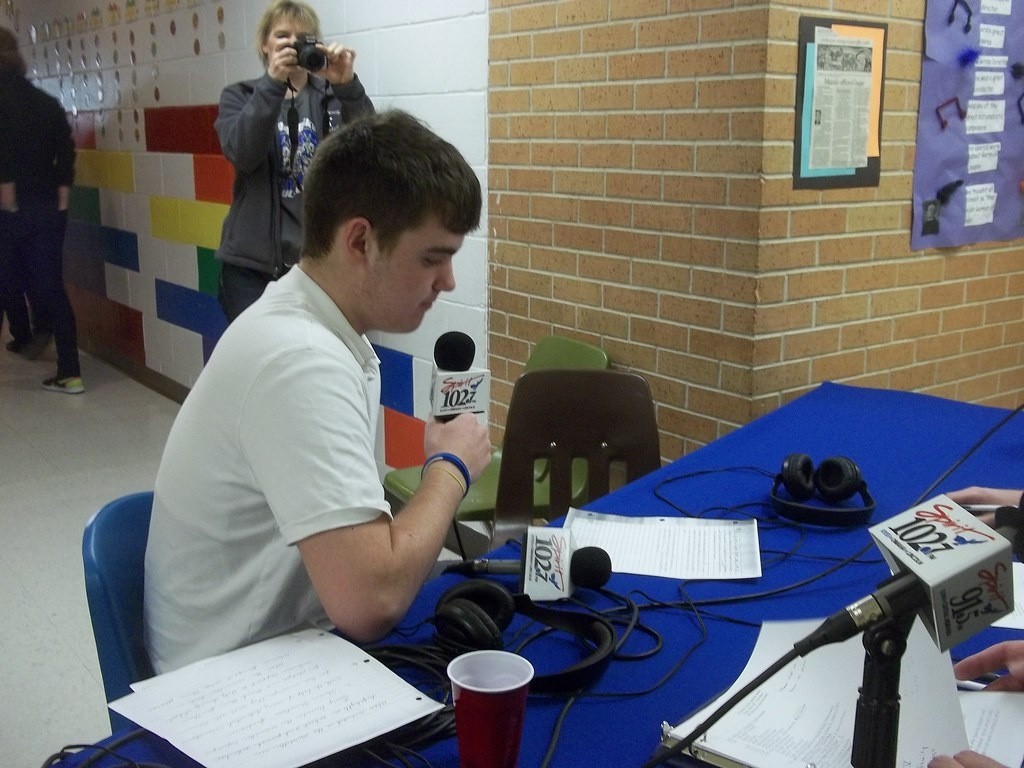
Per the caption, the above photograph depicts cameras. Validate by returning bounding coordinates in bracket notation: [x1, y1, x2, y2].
[288, 34, 328, 74]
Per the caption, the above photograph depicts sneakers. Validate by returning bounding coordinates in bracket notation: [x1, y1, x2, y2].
[44, 375, 82, 393]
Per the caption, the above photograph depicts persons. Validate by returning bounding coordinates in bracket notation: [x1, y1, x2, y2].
[213, 0, 377, 325]
[142, 106, 494, 677]
[0, 25, 86, 396]
[0, 218, 57, 359]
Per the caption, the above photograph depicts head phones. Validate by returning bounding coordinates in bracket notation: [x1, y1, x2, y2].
[432, 585, 615, 695]
[772, 456, 875, 524]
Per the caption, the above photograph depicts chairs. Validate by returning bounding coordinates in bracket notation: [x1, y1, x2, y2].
[80, 490, 157, 743]
[382, 333, 612, 561]
[483, 366, 663, 574]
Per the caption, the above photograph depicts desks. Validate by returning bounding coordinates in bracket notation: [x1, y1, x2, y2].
[43, 380, 1023, 767]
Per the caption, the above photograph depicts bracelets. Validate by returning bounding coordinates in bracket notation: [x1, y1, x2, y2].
[421, 467, 466, 497]
[421, 451, 471, 498]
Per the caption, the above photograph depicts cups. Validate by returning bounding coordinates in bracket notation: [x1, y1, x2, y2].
[447, 649, 534, 768]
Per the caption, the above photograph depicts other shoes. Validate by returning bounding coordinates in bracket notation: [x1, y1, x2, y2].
[6, 339, 39, 356]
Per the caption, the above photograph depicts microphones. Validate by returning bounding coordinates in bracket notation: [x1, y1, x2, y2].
[429, 332, 492, 425]
[798, 494, 1023, 659]
[446, 525, 613, 602]
